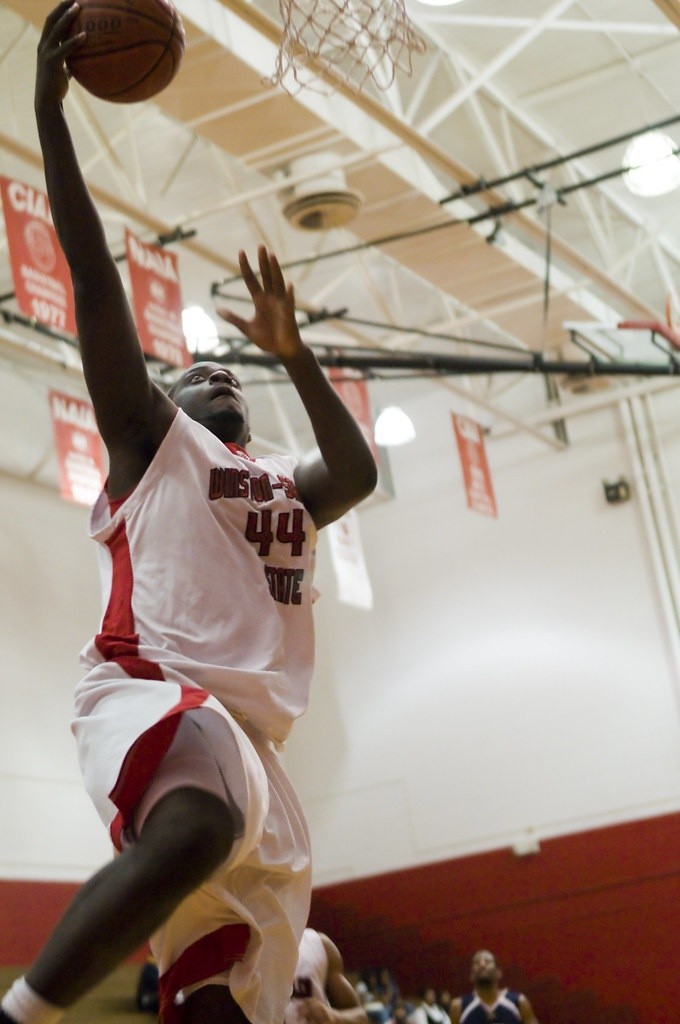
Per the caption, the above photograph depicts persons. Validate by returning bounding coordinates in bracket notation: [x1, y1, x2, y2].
[0, 1, 377, 1024]
[282, 927, 539, 1024]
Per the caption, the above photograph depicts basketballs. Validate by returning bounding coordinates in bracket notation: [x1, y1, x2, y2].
[62, 0, 186, 104]
